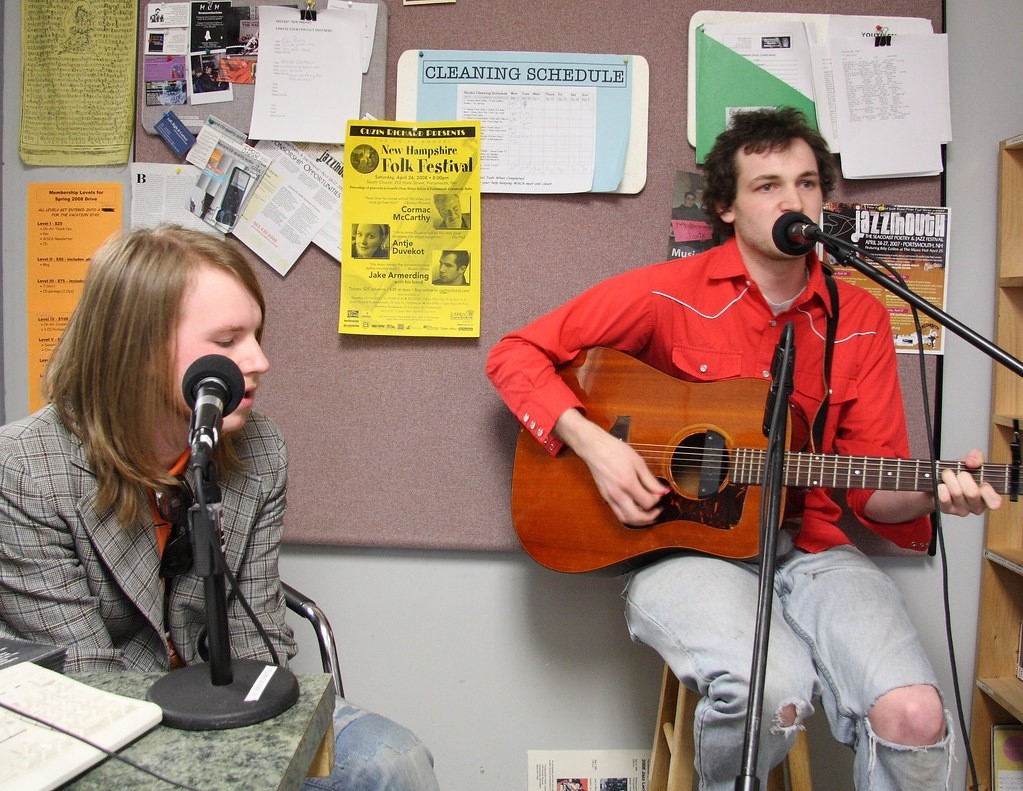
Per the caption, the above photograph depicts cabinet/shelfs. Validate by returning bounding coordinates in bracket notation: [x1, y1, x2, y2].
[964, 134, 1023, 791]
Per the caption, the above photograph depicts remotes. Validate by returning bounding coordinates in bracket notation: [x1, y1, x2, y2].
[216, 166, 251, 226]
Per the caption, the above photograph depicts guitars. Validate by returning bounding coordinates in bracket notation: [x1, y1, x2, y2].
[511, 349, 1022, 575]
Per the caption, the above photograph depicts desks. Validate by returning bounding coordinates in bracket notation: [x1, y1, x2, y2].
[57, 669, 336, 790]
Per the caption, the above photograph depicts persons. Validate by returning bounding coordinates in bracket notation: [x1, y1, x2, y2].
[150, 8, 163, 22]
[483, 104, 1005, 791]
[193, 64, 228, 93]
[667, 184, 720, 261]
[433, 194, 470, 230]
[352, 224, 390, 258]
[0, 221, 440, 791]
[432, 250, 470, 286]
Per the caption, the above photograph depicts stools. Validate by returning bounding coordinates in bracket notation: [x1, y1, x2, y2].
[645, 661, 815, 791]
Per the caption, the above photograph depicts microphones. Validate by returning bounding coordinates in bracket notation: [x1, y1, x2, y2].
[182, 354, 245, 478]
[772, 211, 822, 256]
